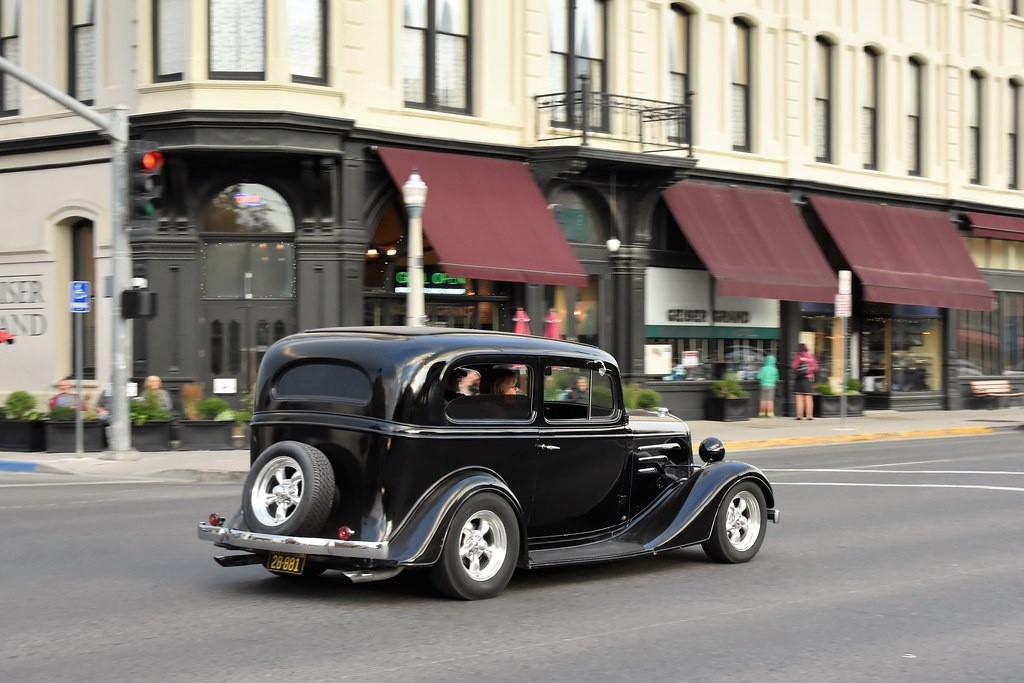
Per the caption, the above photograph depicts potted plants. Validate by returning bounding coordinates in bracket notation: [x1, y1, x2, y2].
[0, 391, 47, 452]
[811, 378, 867, 418]
[177, 398, 234, 450]
[129, 385, 174, 452]
[45, 406, 109, 453]
[708, 373, 752, 422]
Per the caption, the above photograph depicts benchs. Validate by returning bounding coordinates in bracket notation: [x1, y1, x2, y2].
[969, 380, 1024, 410]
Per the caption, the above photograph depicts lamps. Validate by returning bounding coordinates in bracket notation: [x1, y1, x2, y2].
[605, 178, 621, 252]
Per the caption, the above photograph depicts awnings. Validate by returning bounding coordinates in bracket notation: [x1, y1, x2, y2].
[961, 211, 1024, 242]
[805, 194, 993, 312]
[377, 145, 589, 288]
[661, 182, 839, 303]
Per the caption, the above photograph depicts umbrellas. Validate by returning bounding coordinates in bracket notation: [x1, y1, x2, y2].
[510, 307, 534, 370]
[542, 309, 568, 370]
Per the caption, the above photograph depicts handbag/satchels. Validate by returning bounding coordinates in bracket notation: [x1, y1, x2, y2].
[792, 360, 808, 382]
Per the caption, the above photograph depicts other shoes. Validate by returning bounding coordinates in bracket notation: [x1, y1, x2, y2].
[795, 416, 803, 420]
[758, 411, 766, 417]
[766, 412, 775, 417]
[807, 416, 814, 420]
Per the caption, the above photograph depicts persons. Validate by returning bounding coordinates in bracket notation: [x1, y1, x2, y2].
[792, 343, 819, 421]
[138, 375, 173, 412]
[757, 355, 779, 418]
[449, 364, 592, 420]
[51, 382, 108, 417]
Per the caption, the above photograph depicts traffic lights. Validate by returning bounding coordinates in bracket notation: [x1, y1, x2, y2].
[122, 289, 158, 318]
[128, 140, 165, 217]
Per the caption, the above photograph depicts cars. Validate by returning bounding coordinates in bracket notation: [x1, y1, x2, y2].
[197, 325, 779, 602]
[702, 344, 799, 392]
